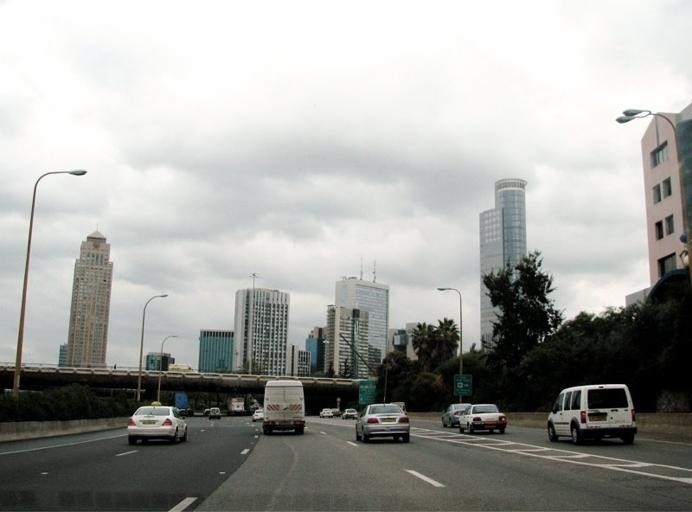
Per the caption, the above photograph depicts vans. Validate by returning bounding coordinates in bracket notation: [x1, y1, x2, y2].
[208, 407, 222, 420]
[546, 383, 638, 447]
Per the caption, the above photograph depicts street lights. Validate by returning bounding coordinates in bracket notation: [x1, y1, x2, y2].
[615, 109, 692, 272]
[137, 294, 168, 409]
[11, 170, 88, 421]
[323, 340, 330, 377]
[157, 335, 178, 406]
[437, 287, 463, 404]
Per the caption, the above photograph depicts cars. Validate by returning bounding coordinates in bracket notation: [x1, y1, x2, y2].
[459, 403, 508, 434]
[177, 407, 195, 418]
[128, 401, 187, 444]
[252, 406, 264, 422]
[203, 409, 210, 416]
[320, 408, 334, 418]
[355, 403, 411, 443]
[341, 408, 358, 420]
[331, 408, 342, 418]
[390, 401, 407, 417]
[441, 402, 472, 428]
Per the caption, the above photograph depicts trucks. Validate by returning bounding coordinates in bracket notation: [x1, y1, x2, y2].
[166, 389, 188, 408]
[227, 397, 245, 416]
[169, 363, 198, 373]
[262, 379, 306, 435]
